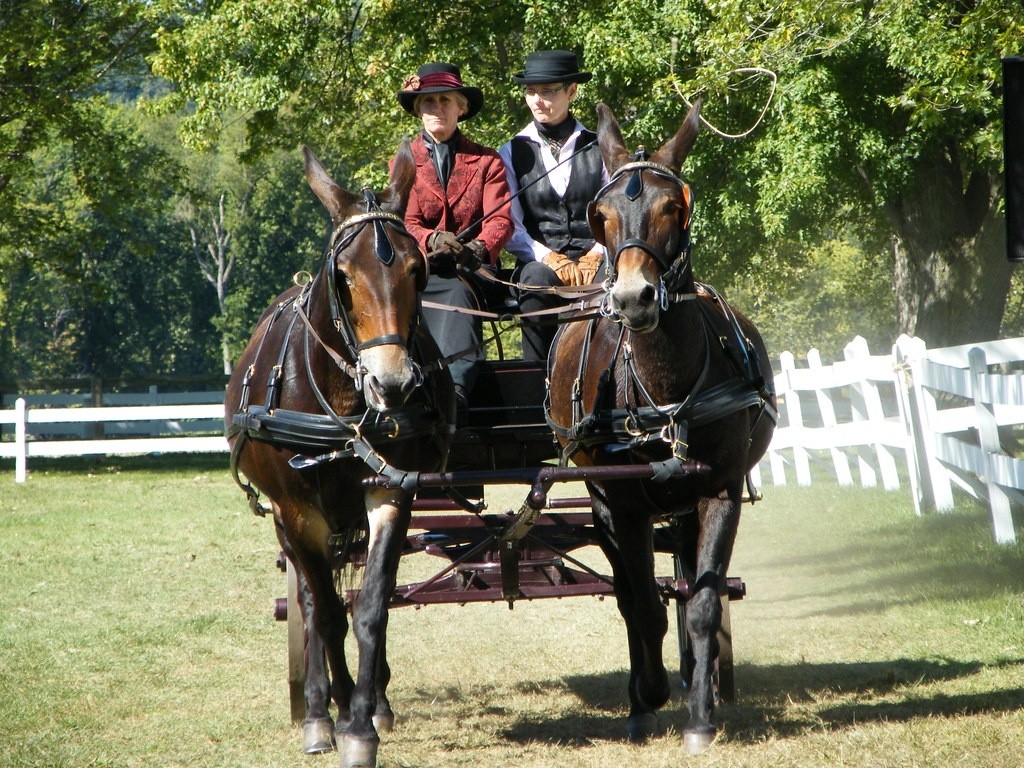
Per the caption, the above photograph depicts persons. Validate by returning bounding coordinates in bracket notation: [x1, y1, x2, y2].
[382, 62, 514, 407]
[499, 50, 611, 359]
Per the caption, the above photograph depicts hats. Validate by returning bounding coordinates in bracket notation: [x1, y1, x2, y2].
[514, 51, 592, 85]
[398, 63, 484, 123]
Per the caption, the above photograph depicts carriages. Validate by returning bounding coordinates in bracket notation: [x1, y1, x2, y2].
[224, 93, 781, 768]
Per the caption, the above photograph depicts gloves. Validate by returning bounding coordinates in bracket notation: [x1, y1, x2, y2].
[577, 250, 604, 286]
[426, 227, 464, 257]
[455, 238, 486, 274]
[542, 251, 583, 293]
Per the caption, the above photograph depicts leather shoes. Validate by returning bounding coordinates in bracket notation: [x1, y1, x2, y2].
[455, 384, 468, 407]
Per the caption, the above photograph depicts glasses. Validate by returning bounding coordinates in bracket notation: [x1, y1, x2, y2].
[524, 84, 569, 95]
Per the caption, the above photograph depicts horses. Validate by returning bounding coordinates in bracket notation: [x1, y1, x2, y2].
[549, 98, 777, 753]
[224, 138, 455, 768]
